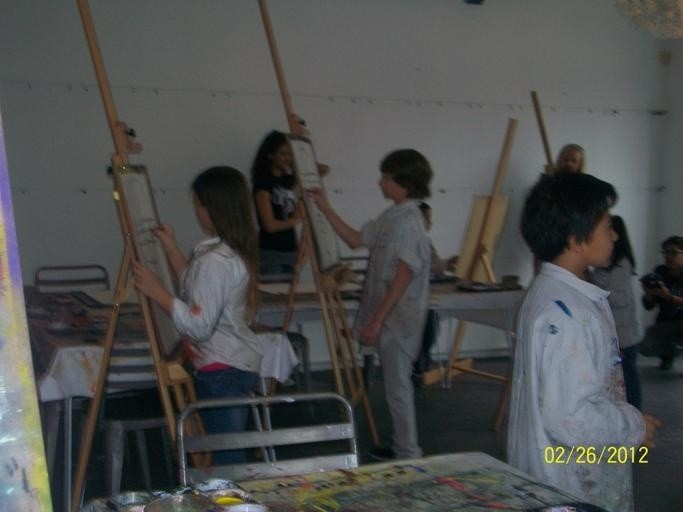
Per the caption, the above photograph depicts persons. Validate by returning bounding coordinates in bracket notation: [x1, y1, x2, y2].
[131, 165, 258, 464]
[595, 213, 643, 413]
[507, 165, 662, 511]
[546, 141, 588, 177]
[636, 235, 683, 368]
[416, 203, 458, 375]
[250, 130, 330, 274]
[308, 148, 437, 461]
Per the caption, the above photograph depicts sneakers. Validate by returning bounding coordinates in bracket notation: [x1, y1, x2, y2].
[659, 357, 674, 369]
[369, 448, 395, 461]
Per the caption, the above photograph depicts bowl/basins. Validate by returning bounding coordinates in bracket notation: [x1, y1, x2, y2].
[109, 488, 155, 511]
